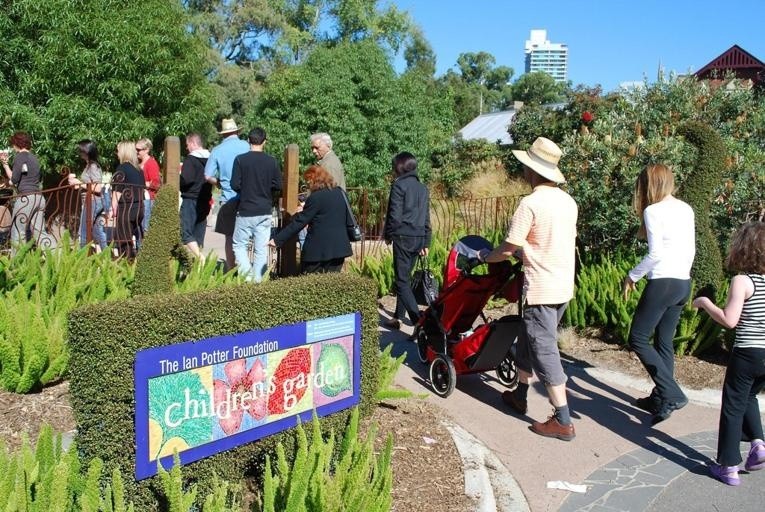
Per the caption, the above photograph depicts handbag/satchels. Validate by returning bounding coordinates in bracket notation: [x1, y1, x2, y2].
[336, 187, 362, 242]
[409, 257, 436, 306]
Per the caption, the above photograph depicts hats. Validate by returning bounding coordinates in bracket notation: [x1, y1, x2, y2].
[512, 137, 567, 185]
[219, 119, 244, 134]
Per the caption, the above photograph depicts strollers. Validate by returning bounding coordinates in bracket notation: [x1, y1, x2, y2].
[413, 235, 523, 395]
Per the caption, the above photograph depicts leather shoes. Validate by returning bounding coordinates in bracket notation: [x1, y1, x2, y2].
[529, 418, 577, 442]
[500, 390, 528, 416]
[407, 324, 424, 342]
[383, 320, 400, 330]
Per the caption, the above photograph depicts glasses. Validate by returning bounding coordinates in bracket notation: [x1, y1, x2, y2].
[137, 148, 148, 153]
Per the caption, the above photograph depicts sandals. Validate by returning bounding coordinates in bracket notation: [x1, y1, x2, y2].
[744, 442, 765, 470]
[710, 464, 741, 487]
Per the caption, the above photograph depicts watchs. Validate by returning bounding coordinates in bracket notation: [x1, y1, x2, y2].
[483, 251, 489, 263]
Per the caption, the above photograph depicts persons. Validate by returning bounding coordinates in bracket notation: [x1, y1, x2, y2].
[135, 137, 160, 248]
[204, 118, 251, 270]
[0, 198, 12, 233]
[382, 151, 432, 327]
[267, 165, 353, 275]
[310, 132, 346, 192]
[0, 132, 48, 257]
[622, 163, 696, 426]
[230, 126, 282, 283]
[68, 139, 108, 255]
[180, 132, 213, 257]
[691, 221, 765, 486]
[111, 141, 145, 258]
[476, 136, 578, 442]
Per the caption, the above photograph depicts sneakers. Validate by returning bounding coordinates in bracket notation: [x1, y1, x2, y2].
[650, 400, 690, 427]
[635, 395, 663, 412]
